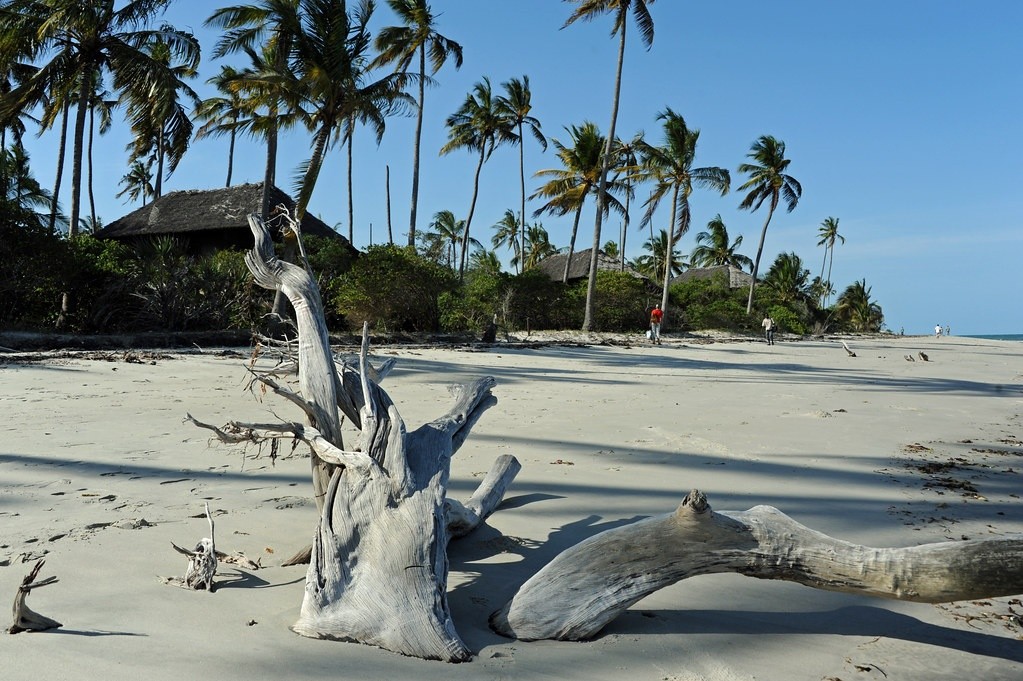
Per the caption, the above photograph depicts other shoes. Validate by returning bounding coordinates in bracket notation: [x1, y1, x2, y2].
[658, 343, 661, 345]
[772, 343, 774, 345]
[652, 342, 655, 344]
[767, 343, 770, 345]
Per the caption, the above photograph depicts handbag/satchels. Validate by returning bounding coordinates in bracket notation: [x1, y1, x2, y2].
[646, 330, 651, 340]
[772, 325, 778, 332]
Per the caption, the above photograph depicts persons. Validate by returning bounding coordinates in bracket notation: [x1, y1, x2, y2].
[649, 304, 664, 345]
[935, 324, 950, 337]
[762, 314, 775, 345]
[901, 327, 904, 335]
